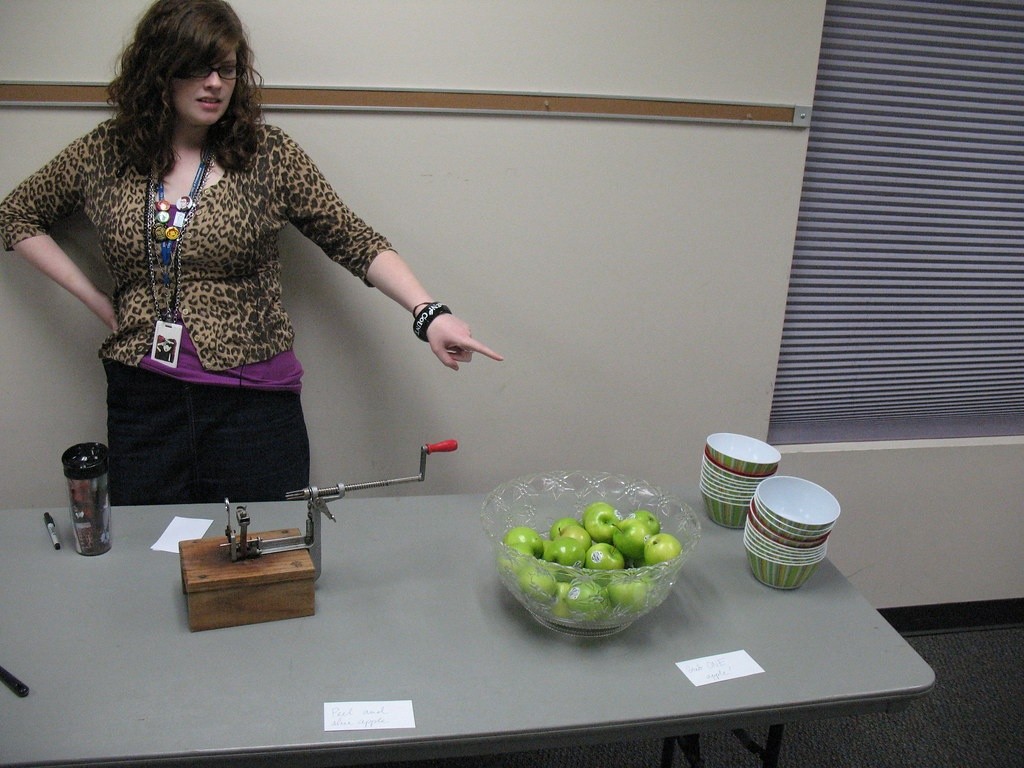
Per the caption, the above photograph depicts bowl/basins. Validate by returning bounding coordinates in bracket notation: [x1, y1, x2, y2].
[479, 471, 699, 639]
[699, 433, 782, 529]
[743, 476, 841, 590]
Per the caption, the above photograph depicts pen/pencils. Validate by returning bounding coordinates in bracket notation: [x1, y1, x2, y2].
[44, 512, 60, 550]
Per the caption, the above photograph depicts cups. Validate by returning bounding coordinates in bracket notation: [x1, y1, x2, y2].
[63, 442, 113, 556]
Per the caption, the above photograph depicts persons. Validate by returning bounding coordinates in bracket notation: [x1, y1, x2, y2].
[0, 0, 503, 506]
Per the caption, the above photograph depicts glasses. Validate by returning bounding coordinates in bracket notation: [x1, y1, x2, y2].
[188, 63, 243, 80]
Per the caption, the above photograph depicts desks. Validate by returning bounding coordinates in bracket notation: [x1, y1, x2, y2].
[0, 490, 937, 768]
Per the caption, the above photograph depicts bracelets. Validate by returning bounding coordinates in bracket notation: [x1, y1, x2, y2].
[413, 302, 431, 318]
[413, 302, 453, 342]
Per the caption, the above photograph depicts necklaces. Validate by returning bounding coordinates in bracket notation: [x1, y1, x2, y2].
[147, 148, 214, 326]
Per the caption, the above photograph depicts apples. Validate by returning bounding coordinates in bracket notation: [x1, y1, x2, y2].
[497, 501, 682, 620]
